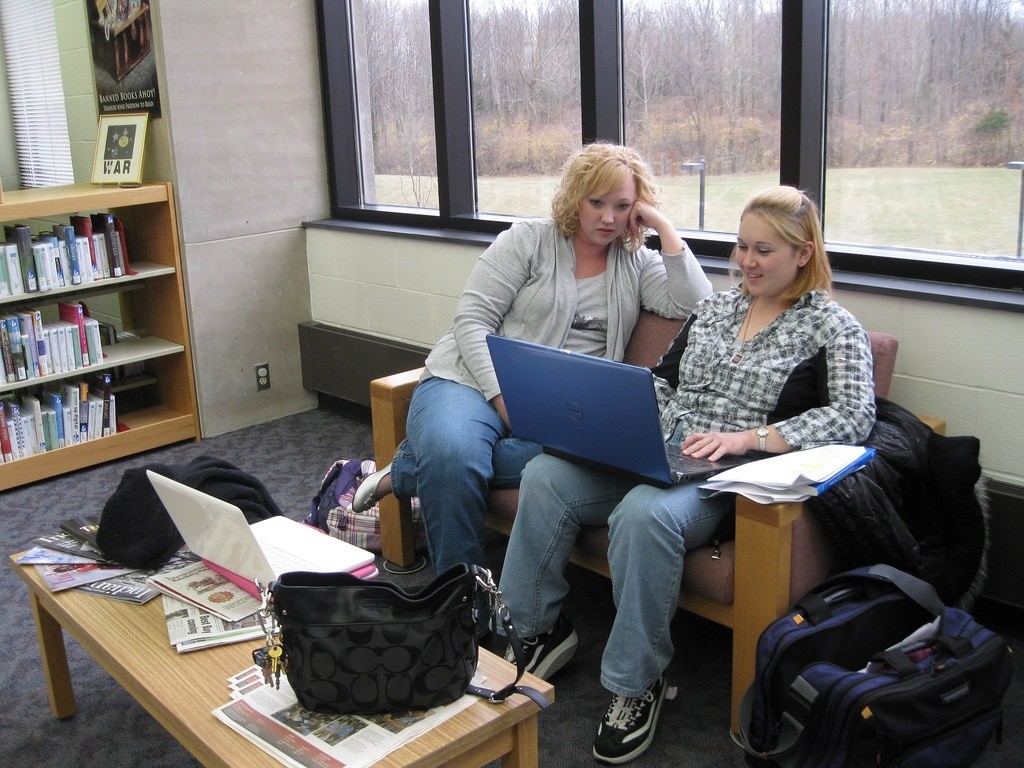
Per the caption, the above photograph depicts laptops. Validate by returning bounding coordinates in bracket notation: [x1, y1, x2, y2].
[485, 334, 758, 485]
[145, 469, 376, 590]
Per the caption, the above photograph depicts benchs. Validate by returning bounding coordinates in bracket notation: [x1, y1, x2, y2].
[371, 311, 946, 736]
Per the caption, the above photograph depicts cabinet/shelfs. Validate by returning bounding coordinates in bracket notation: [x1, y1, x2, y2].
[0, 181, 220, 492]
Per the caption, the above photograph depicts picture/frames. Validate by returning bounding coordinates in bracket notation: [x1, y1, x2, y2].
[90, 112, 151, 189]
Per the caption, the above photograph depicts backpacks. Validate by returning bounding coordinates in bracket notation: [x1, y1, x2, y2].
[303, 458, 428, 553]
[729, 562, 1015, 768]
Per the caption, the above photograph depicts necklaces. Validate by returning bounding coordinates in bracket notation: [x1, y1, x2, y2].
[730, 296, 784, 366]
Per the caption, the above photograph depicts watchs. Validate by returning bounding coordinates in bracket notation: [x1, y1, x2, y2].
[756, 426, 770, 452]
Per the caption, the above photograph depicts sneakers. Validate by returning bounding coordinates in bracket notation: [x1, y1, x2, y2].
[503, 610, 578, 681]
[592, 672, 667, 765]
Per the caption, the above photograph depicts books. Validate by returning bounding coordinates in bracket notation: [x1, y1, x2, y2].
[0, 301, 104, 386]
[792, 443, 877, 497]
[0, 370, 117, 464]
[0, 212, 132, 298]
[14, 514, 203, 606]
[99, 320, 145, 379]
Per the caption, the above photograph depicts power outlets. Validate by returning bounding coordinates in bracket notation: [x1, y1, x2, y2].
[253, 361, 272, 393]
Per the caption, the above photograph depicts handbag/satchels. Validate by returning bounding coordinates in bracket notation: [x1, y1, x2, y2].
[268, 562, 549, 716]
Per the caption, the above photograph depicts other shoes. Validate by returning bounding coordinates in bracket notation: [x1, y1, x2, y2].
[353, 460, 394, 513]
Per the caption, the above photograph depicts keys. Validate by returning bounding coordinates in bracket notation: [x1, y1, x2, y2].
[252, 642, 289, 690]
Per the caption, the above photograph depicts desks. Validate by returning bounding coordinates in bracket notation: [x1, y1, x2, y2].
[9, 507, 557, 766]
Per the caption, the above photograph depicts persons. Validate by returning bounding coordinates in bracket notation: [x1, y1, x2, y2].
[276, 707, 429, 746]
[496, 185, 877, 764]
[353, 140, 713, 636]
